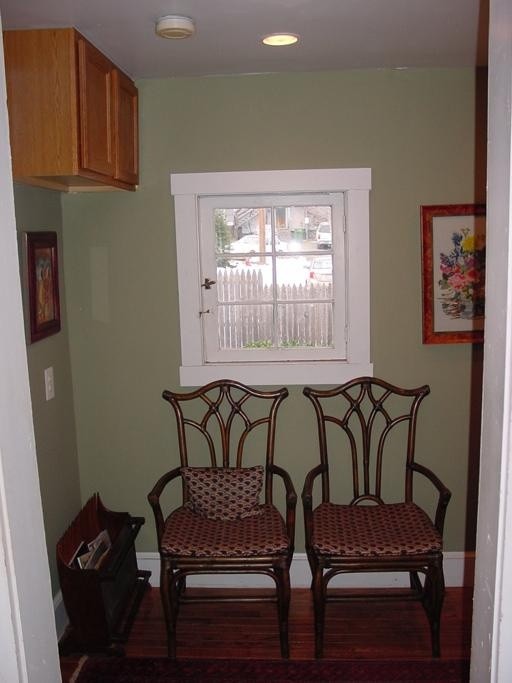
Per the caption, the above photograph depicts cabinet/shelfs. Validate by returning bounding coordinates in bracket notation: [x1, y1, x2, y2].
[2, 27, 140, 192]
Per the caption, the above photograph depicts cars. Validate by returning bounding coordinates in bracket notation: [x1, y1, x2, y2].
[256, 222, 279, 236]
[304, 256, 333, 285]
[224, 234, 288, 256]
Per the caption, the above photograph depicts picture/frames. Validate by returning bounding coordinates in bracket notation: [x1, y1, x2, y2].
[21, 231, 61, 346]
[420, 204, 472, 345]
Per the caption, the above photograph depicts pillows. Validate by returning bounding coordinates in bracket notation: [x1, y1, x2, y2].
[179, 464, 265, 522]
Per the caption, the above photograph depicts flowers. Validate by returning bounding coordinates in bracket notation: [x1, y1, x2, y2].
[438, 227, 473, 319]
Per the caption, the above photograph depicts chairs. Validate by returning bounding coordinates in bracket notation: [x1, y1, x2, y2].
[148, 378, 298, 661]
[303, 376, 452, 658]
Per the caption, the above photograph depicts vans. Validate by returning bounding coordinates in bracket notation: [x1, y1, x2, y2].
[314, 222, 332, 249]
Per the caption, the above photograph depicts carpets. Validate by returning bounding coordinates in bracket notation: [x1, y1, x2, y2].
[67, 656, 470, 683]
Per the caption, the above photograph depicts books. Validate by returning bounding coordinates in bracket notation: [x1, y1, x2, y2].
[68, 529, 112, 570]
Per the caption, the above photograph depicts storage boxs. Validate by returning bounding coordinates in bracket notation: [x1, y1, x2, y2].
[55, 492, 152, 657]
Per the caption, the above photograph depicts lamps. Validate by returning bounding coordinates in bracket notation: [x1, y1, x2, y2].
[155, 14, 195, 39]
